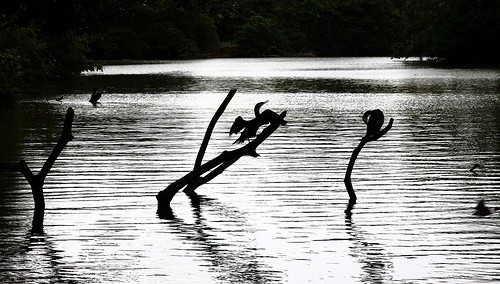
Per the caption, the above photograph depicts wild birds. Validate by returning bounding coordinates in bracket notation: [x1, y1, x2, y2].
[229, 100, 287, 146]
[89, 88, 106, 107]
[473, 198, 499, 216]
[362, 108, 385, 137]
